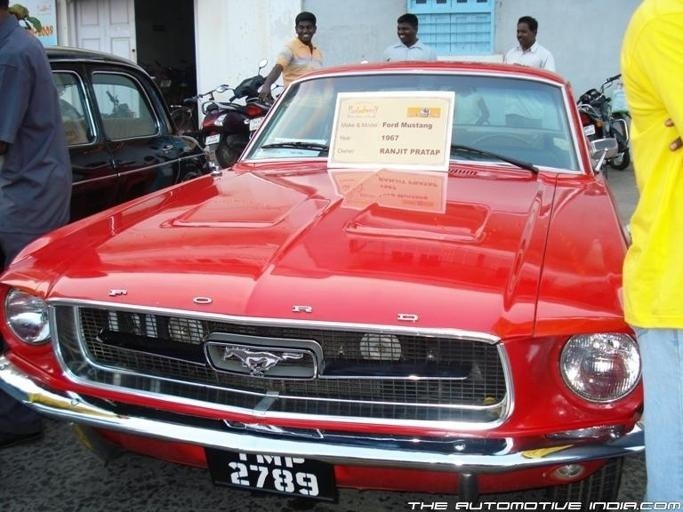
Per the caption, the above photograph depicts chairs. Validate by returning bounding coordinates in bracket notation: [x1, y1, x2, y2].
[100, 117, 155, 140]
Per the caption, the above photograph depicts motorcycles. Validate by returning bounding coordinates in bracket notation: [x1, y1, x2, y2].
[155, 59, 178, 100]
[576, 74, 630, 178]
[201, 59, 276, 168]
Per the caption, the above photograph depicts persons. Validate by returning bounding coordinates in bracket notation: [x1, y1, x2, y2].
[258, 12, 324, 102]
[1, 1, 73, 449]
[386, 13, 437, 61]
[453, 85, 490, 125]
[504, 15, 554, 127]
[621, 0, 682, 512]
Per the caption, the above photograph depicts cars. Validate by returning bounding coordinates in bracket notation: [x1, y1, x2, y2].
[44, 46, 218, 223]
[0, 60, 646, 508]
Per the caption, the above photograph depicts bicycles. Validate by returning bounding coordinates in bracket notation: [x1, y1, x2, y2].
[168, 86, 227, 148]
